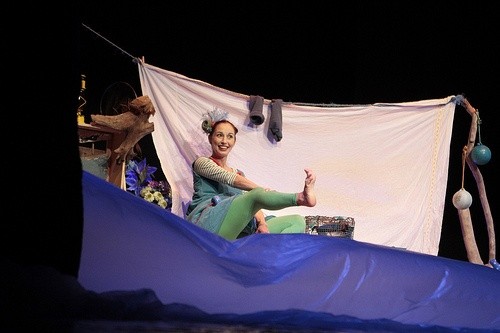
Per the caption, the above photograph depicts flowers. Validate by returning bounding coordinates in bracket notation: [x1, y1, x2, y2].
[123, 155, 172, 212]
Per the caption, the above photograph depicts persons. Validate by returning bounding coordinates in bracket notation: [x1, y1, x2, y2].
[184, 117, 318, 240]
